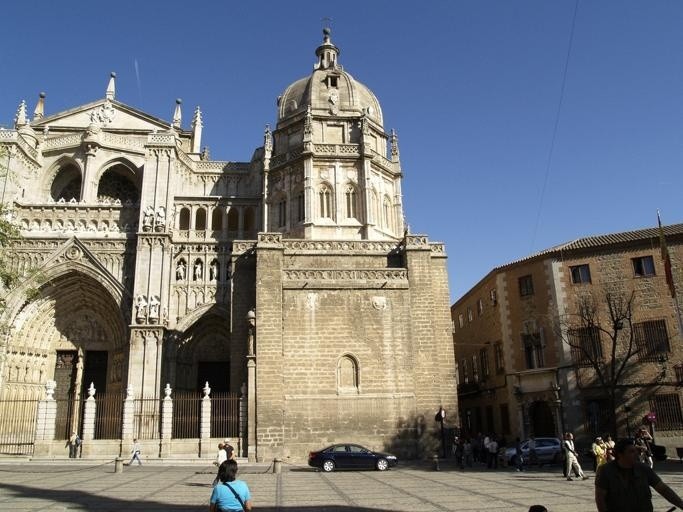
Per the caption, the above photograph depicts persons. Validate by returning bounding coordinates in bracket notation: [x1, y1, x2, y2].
[136, 295, 148, 318]
[69, 431, 78, 458]
[176, 261, 186, 282]
[451, 426, 507, 469]
[526, 433, 544, 469]
[142, 206, 155, 226]
[210, 262, 219, 280]
[595, 438, 682, 512]
[223, 439, 235, 460]
[208, 443, 227, 488]
[528, 505, 547, 512]
[126, 439, 142, 467]
[149, 295, 160, 317]
[155, 205, 165, 226]
[591, 427, 653, 472]
[209, 459, 252, 512]
[515, 437, 527, 472]
[558, 432, 589, 481]
[195, 263, 202, 280]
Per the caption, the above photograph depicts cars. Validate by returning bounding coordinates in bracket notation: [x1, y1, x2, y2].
[497, 437, 562, 467]
[308, 443, 398, 472]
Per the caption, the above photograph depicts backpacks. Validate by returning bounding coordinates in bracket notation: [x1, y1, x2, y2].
[76, 437, 81, 446]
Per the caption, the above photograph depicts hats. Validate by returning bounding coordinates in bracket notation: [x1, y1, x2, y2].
[224, 438, 230, 443]
